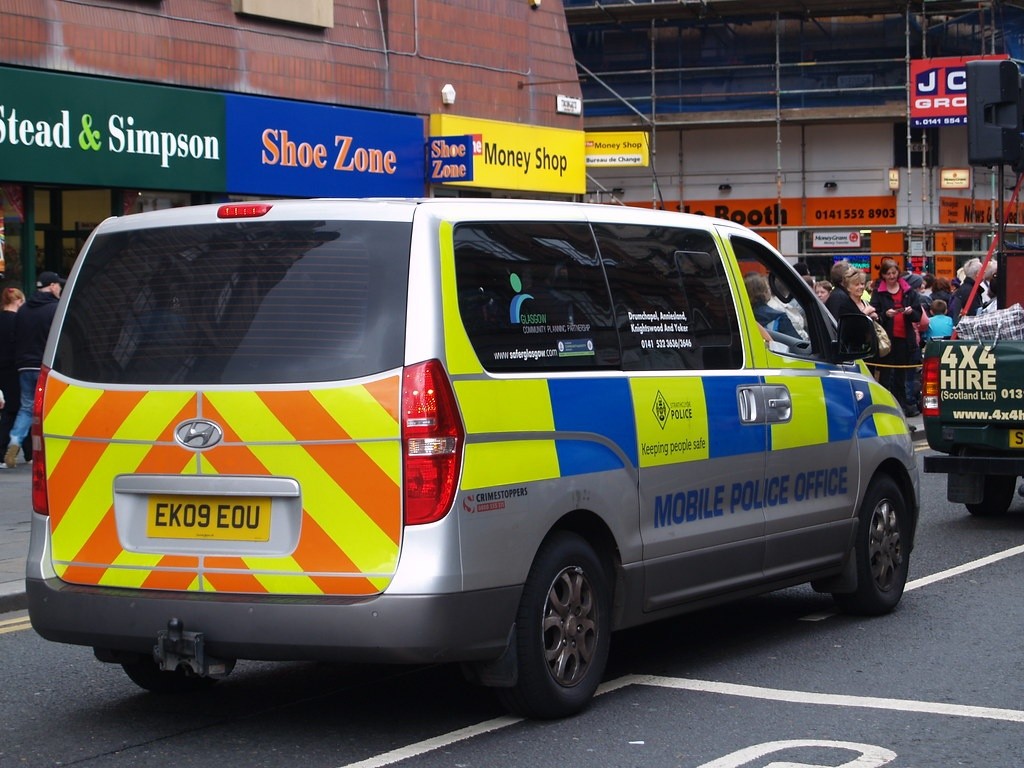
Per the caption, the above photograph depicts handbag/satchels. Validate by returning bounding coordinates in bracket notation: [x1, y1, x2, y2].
[958, 302, 1023, 351]
[872, 320, 892, 356]
[919, 304, 930, 334]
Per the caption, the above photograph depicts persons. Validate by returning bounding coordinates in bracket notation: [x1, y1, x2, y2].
[0, 287, 34, 469]
[3, 270, 67, 468]
[741, 256, 1000, 418]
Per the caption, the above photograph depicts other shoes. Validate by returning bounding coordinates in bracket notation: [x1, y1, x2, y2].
[0, 462, 16, 469]
[4, 444, 20, 468]
[904, 404, 921, 417]
[28, 459, 34, 464]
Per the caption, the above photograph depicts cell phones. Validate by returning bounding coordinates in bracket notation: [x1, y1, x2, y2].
[897, 308, 904, 312]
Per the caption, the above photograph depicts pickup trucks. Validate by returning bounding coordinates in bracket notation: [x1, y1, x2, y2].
[923, 251, 1023, 519]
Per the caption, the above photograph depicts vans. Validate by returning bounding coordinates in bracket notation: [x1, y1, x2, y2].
[25, 193, 923, 723]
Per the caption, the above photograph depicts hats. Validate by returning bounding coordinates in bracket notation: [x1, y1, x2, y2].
[36, 271, 67, 288]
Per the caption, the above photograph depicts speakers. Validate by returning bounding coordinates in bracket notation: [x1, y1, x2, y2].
[965, 59, 1021, 170]
